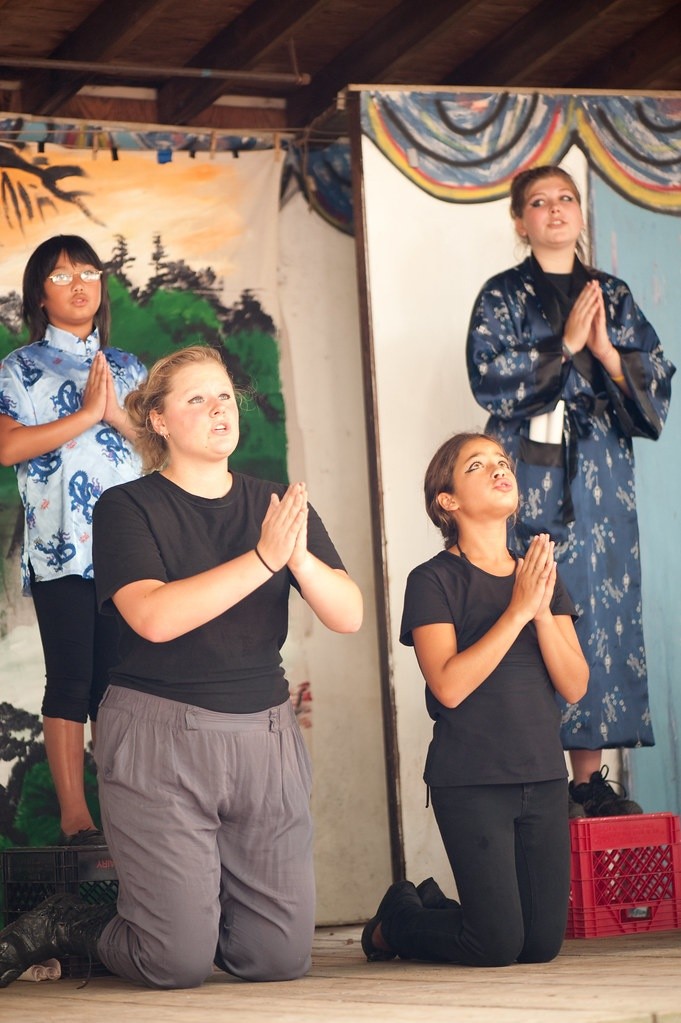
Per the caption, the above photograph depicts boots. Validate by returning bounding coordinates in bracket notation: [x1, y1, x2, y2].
[0, 893, 118, 989]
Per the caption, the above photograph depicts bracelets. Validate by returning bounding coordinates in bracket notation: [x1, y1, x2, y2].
[612, 375, 624, 382]
[563, 344, 572, 363]
[254, 547, 276, 574]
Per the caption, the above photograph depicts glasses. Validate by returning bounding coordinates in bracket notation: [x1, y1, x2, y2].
[46, 269, 103, 285]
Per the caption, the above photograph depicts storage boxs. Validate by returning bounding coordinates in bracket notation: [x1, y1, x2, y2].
[0, 845, 120, 926]
[562, 811, 679, 940]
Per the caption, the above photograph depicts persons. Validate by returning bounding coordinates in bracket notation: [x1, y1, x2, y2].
[467, 165, 675, 816]
[361, 432, 588, 966]
[0, 346, 364, 990]
[0, 238, 147, 850]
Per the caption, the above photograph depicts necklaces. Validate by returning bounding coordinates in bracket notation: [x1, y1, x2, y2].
[457, 541, 518, 576]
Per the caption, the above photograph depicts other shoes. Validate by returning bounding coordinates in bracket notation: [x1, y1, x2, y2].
[58, 825, 107, 846]
[416, 876, 446, 909]
[361, 880, 423, 962]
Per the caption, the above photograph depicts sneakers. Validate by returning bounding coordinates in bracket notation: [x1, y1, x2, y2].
[568, 794, 586, 818]
[568, 765, 643, 816]
